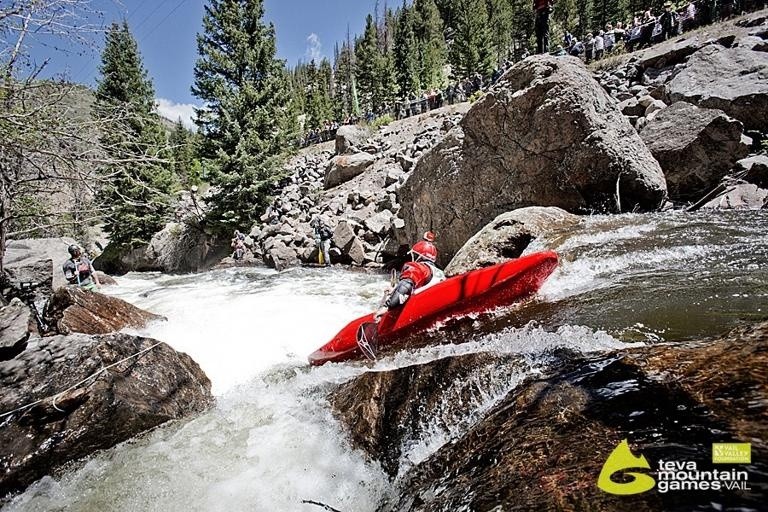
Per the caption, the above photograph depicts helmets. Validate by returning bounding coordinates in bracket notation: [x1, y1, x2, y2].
[411, 241, 437, 263]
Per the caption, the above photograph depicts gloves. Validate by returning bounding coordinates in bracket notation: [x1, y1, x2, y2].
[423, 231, 435, 242]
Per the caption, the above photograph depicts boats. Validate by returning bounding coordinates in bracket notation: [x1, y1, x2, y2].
[308, 251, 559, 368]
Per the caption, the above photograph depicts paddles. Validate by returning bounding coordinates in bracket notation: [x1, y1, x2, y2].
[356, 268, 396, 362]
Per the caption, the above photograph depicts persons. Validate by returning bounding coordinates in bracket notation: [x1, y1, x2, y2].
[531, 1, 768, 62]
[299, 47, 526, 151]
[386, 231, 450, 308]
[62, 244, 102, 292]
